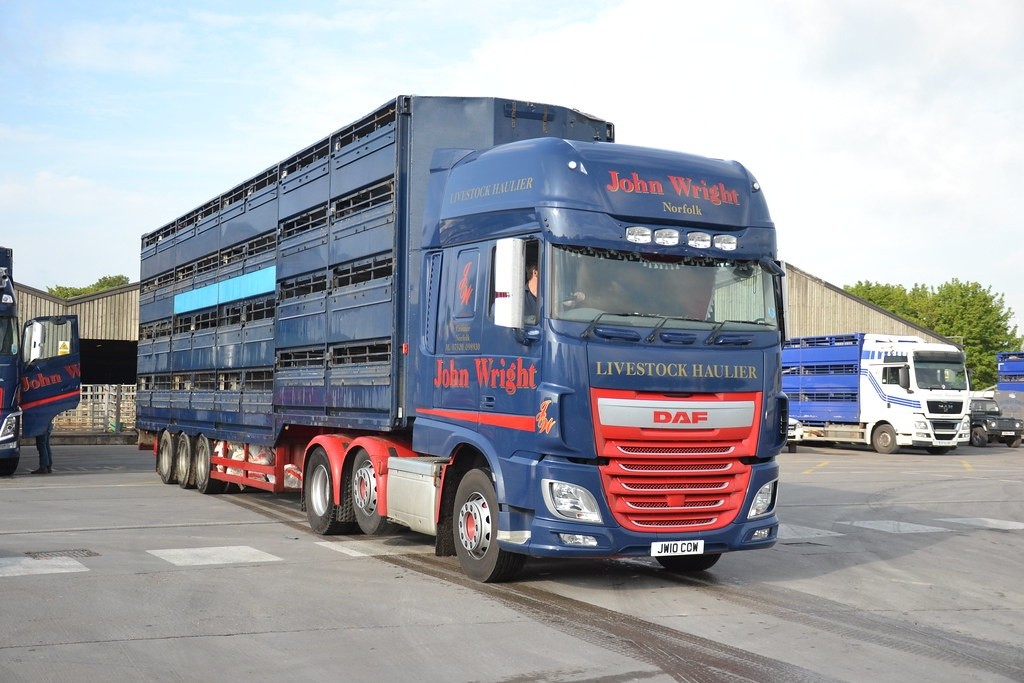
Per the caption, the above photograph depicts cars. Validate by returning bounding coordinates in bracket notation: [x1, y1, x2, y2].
[779, 417, 804, 455]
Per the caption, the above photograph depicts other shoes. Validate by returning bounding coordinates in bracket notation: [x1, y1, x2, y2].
[31, 466, 52, 474]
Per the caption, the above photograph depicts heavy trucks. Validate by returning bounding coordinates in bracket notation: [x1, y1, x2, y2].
[997, 352, 1024, 392]
[136, 93, 790, 585]
[780, 332, 972, 454]
[0, 247, 81, 477]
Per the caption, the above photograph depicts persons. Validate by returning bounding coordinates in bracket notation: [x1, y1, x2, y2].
[489, 262, 587, 324]
[30, 358, 53, 475]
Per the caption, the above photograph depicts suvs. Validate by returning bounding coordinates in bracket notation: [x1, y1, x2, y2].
[970, 397, 1024, 448]
